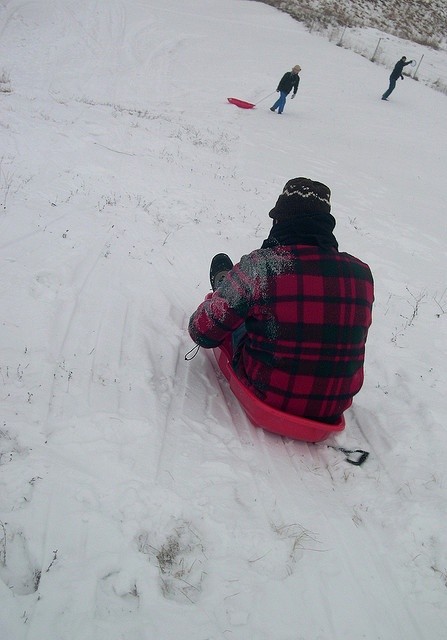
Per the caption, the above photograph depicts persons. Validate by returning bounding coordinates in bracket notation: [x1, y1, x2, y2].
[381, 56, 412, 100]
[187, 176, 372, 421]
[269, 65, 301, 114]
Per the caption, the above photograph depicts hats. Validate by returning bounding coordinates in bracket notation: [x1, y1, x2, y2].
[269, 178, 330, 221]
[294, 65, 300, 71]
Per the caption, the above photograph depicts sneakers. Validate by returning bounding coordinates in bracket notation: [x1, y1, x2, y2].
[210, 254, 233, 292]
[278, 112, 282, 114]
[270, 107, 275, 110]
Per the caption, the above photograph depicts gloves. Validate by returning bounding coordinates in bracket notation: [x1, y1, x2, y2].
[291, 93, 296, 99]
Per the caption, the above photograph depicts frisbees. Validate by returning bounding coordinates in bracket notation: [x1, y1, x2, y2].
[410, 60, 416, 67]
